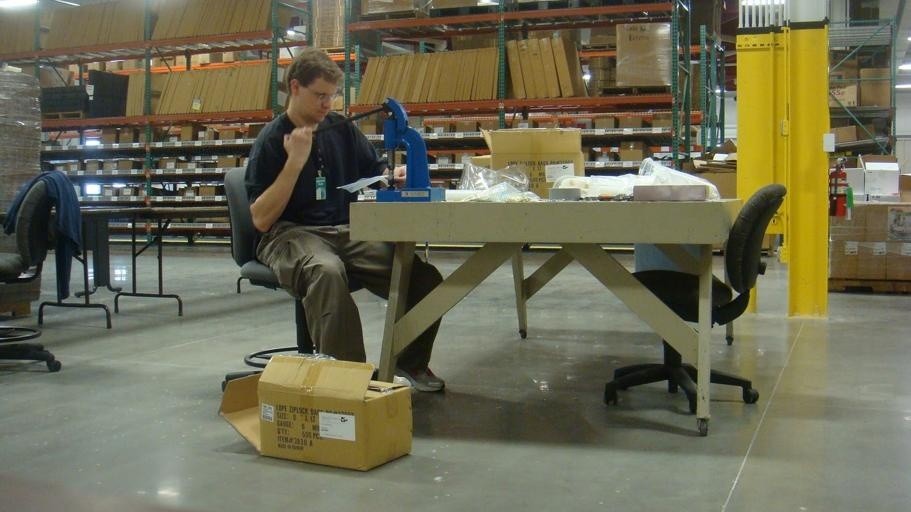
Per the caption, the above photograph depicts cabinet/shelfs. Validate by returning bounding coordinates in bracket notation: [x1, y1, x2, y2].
[1, 0, 314, 244]
[829, 18, 897, 156]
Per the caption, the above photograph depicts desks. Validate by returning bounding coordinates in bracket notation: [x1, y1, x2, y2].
[350, 198, 748, 438]
[1, 206, 232, 329]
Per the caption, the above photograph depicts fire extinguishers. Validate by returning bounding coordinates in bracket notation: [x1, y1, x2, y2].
[829, 156, 849, 217]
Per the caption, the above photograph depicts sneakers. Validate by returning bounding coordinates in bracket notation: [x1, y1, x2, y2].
[395, 363, 445, 393]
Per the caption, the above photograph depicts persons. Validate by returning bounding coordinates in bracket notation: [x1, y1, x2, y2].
[243, 46, 445, 392]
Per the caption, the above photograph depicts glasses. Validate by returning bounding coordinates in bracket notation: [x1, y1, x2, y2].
[301, 79, 338, 104]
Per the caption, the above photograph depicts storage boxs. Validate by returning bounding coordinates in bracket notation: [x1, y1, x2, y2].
[829, 158, 910, 281]
[217, 354, 412, 472]
[832, 66, 894, 152]
[472, 127, 585, 199]
[38, 122, 264, 197]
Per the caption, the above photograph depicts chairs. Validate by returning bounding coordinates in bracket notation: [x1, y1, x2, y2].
[222, 167, 379, 392]
[604, 185, 787, 414]
[0, 171, 68, 372]
